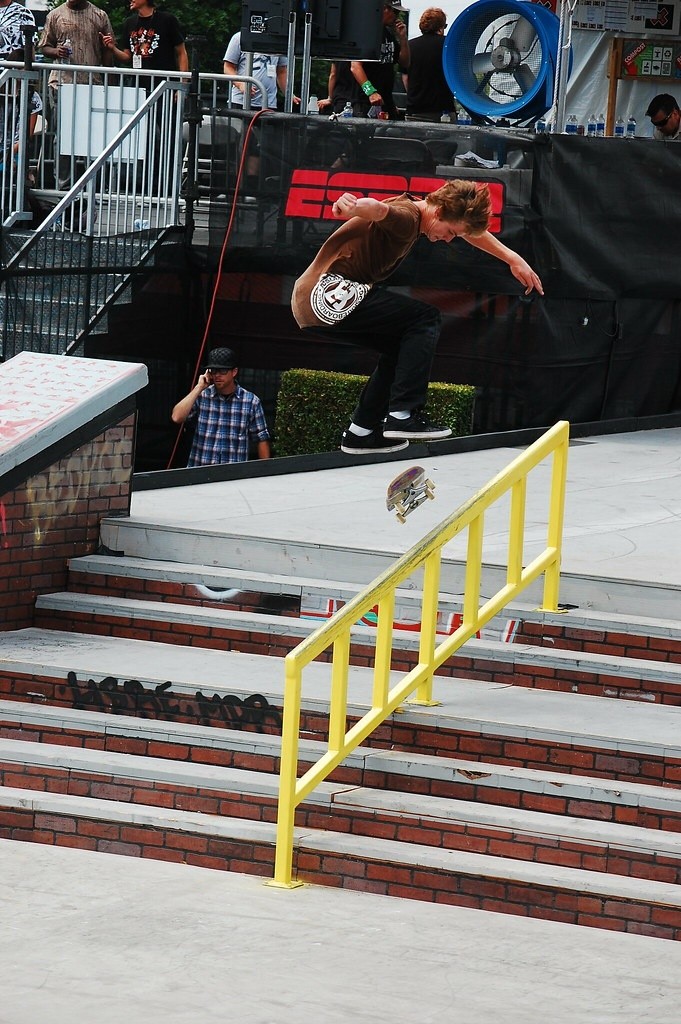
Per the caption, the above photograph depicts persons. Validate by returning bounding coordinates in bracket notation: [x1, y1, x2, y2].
[99, 0, 189, 212]
[317, 61, 351, 114]
[351, 0, 409, 118]
[39, 0, 114, 191]
[223, 31, 302, 204]
[172, 348, 270, 467]
[290, 179, 544, 455]
[0, 0, 42, 157]
[645, 94, 681, 140]
[398, 8, 456, 123]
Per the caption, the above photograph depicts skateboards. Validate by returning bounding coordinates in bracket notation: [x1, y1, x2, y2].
[386, 466, 436, 523]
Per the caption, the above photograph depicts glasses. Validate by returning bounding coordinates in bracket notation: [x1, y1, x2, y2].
[208, 369, 232, 375]
[386, 5, 400, 15]
[651, 110, 677, 126]
[440, 24, 447, 29]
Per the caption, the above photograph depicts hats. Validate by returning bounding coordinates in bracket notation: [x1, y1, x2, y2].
[204, 347, 237, 369]
[384, 0, 409, 11]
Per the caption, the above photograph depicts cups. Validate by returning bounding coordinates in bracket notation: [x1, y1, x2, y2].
[377, 111, 390, 120]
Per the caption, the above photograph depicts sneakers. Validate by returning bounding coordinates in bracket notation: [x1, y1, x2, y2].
[383, 413, 452, 439]
[340, 429, 409, 454]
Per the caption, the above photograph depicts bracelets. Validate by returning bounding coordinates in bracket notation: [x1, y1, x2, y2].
[361, 80, 377, 96]
[328, 96, 334, 102]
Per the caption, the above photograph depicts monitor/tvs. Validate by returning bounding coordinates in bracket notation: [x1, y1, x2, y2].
[240, 0, 385, 62]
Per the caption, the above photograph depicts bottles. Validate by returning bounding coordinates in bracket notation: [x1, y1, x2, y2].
[134, 219, 150, 229]
[457, 109, 472, 138]
[626, 115, 637, 139]
[597, 113, 605, 136]
[587, 114, 596, 137]
[615, 115, 624, 139]
[60, 38, 71, 65]
[441, 110, 451, 124]
[307, 94, 320, 130]
[344, 102, 354, 130]
[536, 114, 578, 136]
